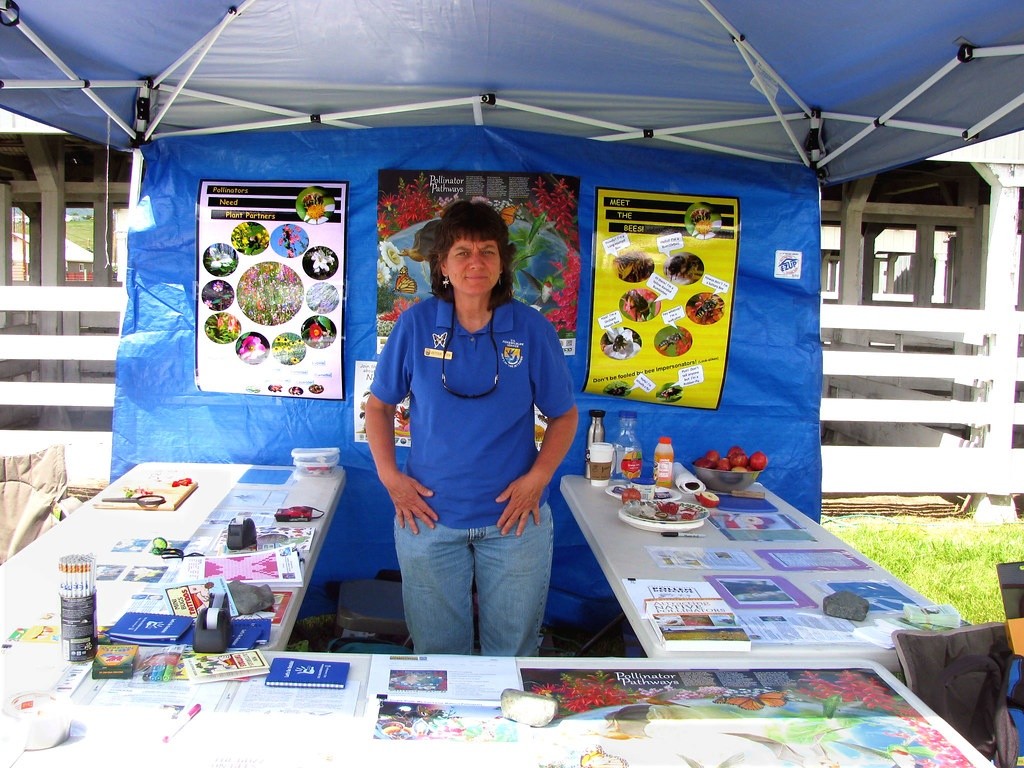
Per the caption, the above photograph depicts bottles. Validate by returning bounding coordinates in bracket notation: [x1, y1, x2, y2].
[585, 410, 606, 479]
[652, 437, 674, 488]
[612, 410, 642, 485]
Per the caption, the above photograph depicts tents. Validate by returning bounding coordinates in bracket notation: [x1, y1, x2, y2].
[0, 2, 1024, 622]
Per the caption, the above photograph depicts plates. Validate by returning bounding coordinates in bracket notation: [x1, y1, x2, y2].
[619, 499, 711, 533]
[605, 484, 682, 503]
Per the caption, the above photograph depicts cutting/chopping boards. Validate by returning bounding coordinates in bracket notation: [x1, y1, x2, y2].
[93, 479, 198, 512]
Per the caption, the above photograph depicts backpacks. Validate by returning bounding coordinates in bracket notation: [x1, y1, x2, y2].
[990, 654, 1024, 768]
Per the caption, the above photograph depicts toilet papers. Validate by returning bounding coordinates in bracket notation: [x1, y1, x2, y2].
[669, 460, 707, 494]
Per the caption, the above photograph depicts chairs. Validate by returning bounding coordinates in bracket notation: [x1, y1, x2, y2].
[891, 622, 1024, 768]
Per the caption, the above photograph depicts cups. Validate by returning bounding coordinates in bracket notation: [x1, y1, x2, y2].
[588, 442, 614, 486]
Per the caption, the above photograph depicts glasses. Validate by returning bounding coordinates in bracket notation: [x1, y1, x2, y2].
[440, 335, 499, 399]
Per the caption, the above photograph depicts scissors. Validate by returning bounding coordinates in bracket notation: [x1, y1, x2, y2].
[102, 495, 166, 510]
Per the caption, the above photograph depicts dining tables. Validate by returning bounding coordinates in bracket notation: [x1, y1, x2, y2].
[559, 473, 976, 674]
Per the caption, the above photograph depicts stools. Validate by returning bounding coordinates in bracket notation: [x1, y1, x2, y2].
[337, 579, 415, 654]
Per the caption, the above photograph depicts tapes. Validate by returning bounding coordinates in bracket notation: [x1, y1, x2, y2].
[206, 607, 220, 630]
[4, 691, 73, 752]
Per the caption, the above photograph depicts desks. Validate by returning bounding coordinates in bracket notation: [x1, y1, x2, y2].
[0, 648, 999, 768]
[1, 461, 350, 652]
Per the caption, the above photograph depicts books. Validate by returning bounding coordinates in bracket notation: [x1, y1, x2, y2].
[265, 658, 350, 689]
[642, 597, 751, 652]
[104, 612, 271, 653]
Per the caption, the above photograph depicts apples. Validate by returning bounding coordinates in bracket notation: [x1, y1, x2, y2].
[694, 491, 719, 508]
[622, 488, 641, 506]
[695, 445, 767, 472]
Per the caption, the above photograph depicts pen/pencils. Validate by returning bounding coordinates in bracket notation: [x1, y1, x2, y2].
[161, 703, 202, 744]
[57, 554, 99, 662]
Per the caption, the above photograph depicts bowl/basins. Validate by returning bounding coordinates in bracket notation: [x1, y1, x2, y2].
[691, 461, 764, 493]
[292, 447, 340, 478]
